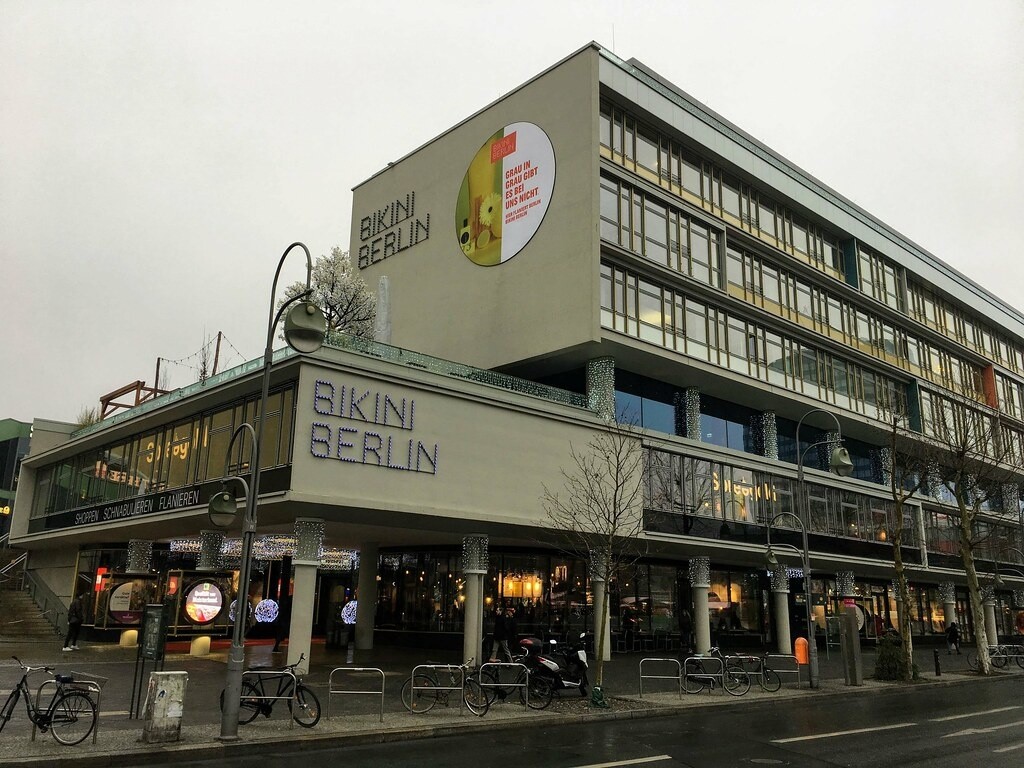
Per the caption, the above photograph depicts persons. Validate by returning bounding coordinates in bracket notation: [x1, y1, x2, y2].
[272, 612, 286, 653]
[490, 608, 513, 664]
[875, 614, 885, 638]
[507, 608, 516, 662]
[944, 622, 962, 655]
[323, 602, 346, 649]
[449, 597, 542, 630]
[62, 591, 84, 651]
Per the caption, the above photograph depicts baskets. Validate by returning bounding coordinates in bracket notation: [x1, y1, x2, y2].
[70, 671, 108, 691]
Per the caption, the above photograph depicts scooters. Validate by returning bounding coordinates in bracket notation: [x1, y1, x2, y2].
[517, 633, 589, 698]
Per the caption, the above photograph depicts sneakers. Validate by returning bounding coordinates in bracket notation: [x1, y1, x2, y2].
[71, 645, 80, 649]
[62, 646, 72, 651]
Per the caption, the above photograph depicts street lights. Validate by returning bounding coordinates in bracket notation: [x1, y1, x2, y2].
[765, 409, 854, 687]
[210, 241, 325, 742]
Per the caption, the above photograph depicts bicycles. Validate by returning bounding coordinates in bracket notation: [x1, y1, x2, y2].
[400, 657, 489, 717]
[464, 647, 553, 709]
[967, 642, 1024, 670]
[717, 651, 782, 692]
[677, 647, 752, 697]
[221, 652, 321, 729]
[0, 655, 109, 745]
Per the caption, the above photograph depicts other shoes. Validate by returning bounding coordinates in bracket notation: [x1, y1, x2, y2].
[273, 650, 283, 653]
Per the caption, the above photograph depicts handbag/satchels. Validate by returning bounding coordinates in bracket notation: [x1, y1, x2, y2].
[70, 617, 84, 625]
[952, 643, 956, 650]
